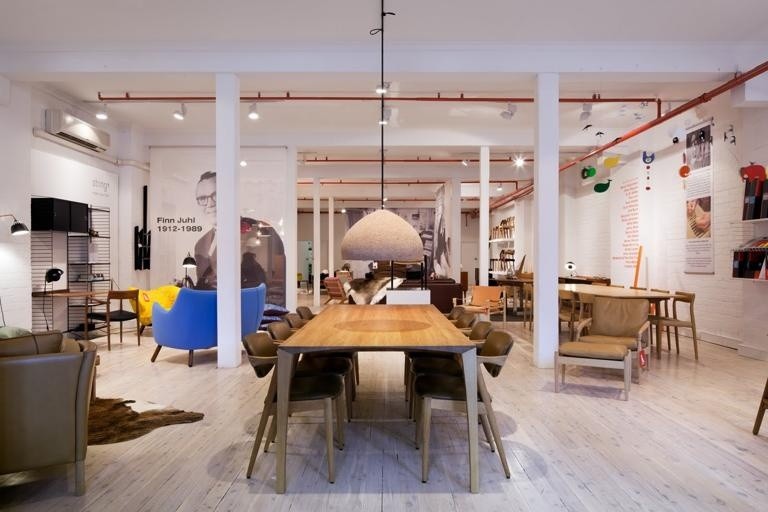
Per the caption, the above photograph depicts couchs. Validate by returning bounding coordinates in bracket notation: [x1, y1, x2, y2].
[0, 338, 97, 495]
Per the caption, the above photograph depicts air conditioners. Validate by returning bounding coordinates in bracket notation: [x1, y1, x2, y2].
[46, 109, 111, 153]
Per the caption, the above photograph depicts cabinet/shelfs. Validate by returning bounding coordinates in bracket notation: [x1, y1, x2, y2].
[67, 208, 117, 331]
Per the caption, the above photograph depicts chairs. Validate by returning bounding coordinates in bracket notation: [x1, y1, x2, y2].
[151, 283, 266, 366]
[127, 285, 180, 333]
[85, 289, 140, 351]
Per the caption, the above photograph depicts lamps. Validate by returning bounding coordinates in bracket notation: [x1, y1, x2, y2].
[564, 262, 576, 272]
[182, 252, 197, 287]
[43, 269, 64, 331]
[0, 214, 29, 326]
[341, 1, 424, 261]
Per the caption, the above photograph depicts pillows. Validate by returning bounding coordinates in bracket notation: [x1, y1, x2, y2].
[0, 327, 32, 339]
[0, 333, 62, 357]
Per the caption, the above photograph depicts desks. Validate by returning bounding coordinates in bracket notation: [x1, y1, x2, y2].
[276, 304, 479, 493]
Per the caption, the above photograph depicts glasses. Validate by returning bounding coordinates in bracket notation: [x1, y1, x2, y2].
[195, 192, 217, 206]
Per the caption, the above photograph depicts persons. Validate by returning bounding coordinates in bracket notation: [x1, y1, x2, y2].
[194, 170, 286, 310]
[687, 199, 711, 231]
